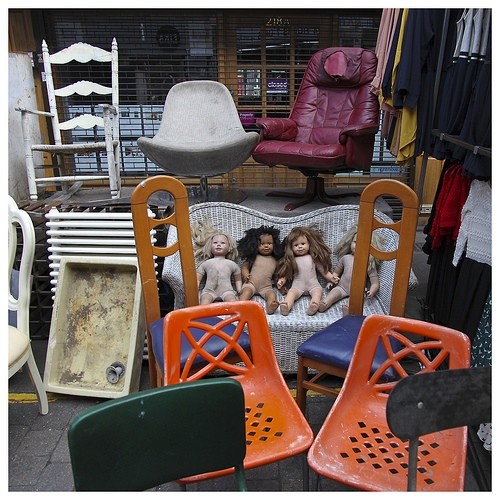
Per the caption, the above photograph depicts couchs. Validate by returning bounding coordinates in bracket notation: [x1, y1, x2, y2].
[162, 200, 418, 375]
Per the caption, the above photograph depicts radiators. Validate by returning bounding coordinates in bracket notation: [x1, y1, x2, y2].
[45, 206, 159, 360]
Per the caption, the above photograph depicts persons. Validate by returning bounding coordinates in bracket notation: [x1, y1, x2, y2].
[317, 226, 382, 316]
[236, 225, 281, 314]
[275, 226, 340, 316]
[193, 221, 243, 305]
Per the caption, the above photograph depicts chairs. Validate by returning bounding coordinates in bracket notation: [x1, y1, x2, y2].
[137, 80, 260, 202]
[14, 36, 122, 202]
[308, 313, 471, 491]
[8, 195, 49, 415]
[252, 47, 380, 211]
[161, 300, 314, 484]
[67, 377, 251, 492]
[385, 366, 492, 493]
[295, 179, 427, 421]
[132, 175, 253, 388]
